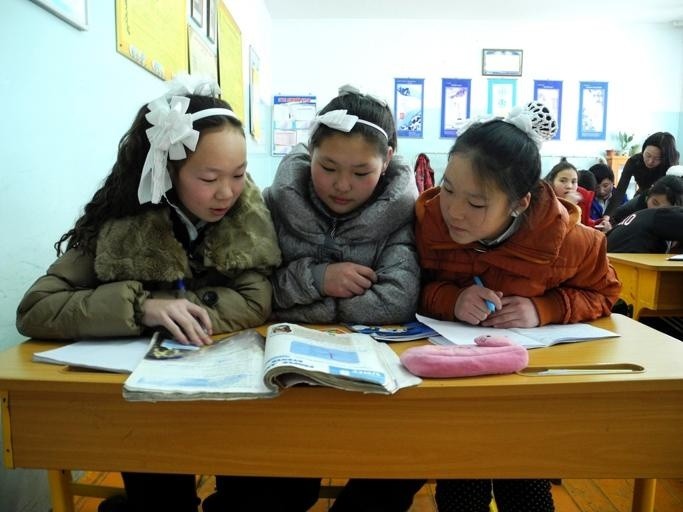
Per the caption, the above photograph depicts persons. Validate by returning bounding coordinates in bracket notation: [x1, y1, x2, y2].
[16, 93, 273, 512]
[415, 116, 620, 512]
[604, 207, 683, 341]
[543, 157, 612, 234]
[609, 175, 683, 227]
[610, 132, 680, 219]
[272, 89, 427, 512]
[589, 164, 627, 218]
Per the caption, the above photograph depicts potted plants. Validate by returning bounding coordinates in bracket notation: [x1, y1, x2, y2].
[616, 131, 635, 156]
[629, 144, 640, 156]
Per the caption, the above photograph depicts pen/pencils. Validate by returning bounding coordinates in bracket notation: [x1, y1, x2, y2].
[473, 275, 494, 317]
[173, 275, 193, 322]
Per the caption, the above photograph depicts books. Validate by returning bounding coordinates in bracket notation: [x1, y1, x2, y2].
[122, 323, 422, 402]
[342, 321, 442, 343]
[666, 254, 683, 260]
[32, 338, 152, 375]
[415, 313, 620, 350]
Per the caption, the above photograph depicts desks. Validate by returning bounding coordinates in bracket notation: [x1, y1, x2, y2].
[0, 312, 683, 512]
[606, 253, 683, 321]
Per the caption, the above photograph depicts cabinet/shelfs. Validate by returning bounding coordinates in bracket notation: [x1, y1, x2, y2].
[598, 155, 640, 191]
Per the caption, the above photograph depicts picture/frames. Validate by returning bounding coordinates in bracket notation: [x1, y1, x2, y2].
[28, 0, 91, 32]
[482, 49, 523, 76]
[191, 0, 203, 29]
[207, 0, 215, 44]
